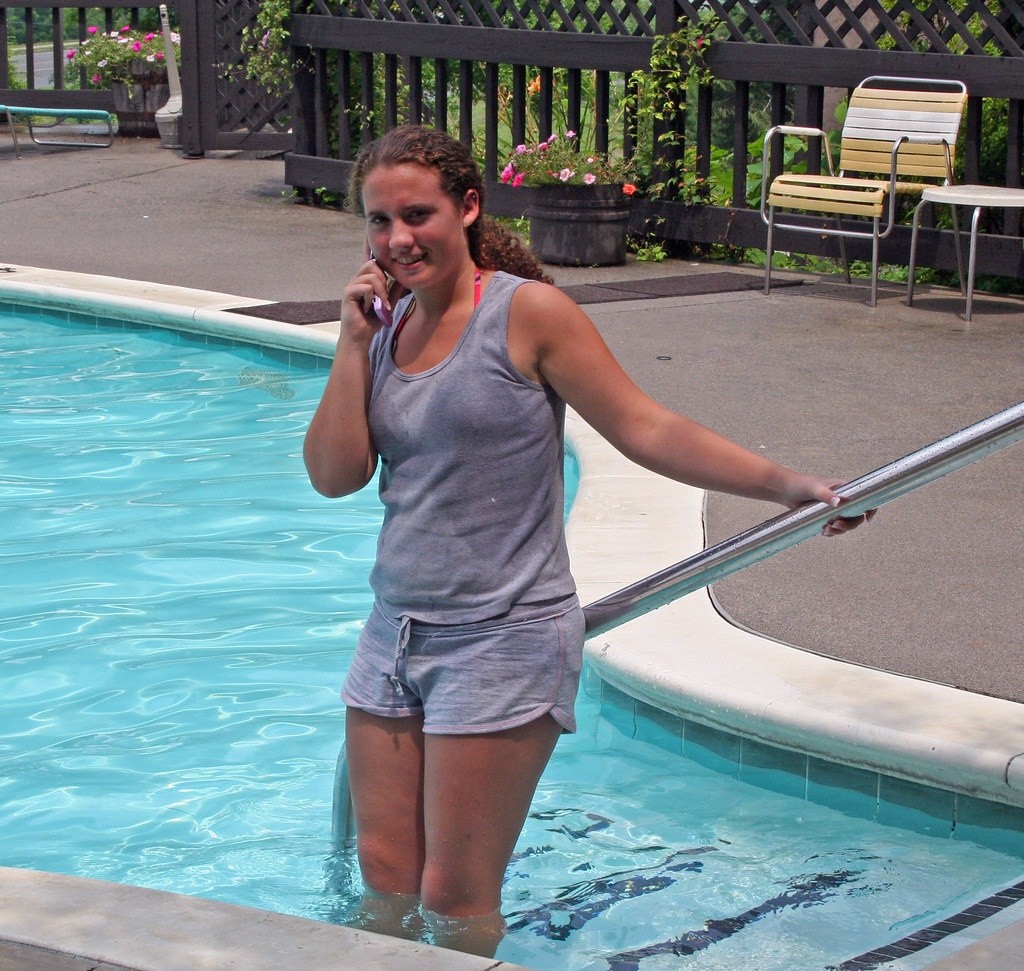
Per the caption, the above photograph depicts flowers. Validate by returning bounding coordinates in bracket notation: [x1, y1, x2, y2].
[67, 23, 183, 84]
[500, 132, 639, 196]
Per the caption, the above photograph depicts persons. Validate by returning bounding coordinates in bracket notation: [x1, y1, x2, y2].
[302, 126, 876, 918]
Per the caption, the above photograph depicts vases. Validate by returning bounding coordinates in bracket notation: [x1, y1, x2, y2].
[112, 59, 181, 140]
[527, 184, 630, 266]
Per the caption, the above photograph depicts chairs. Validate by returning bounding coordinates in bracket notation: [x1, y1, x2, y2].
[760, 76, 968, 307]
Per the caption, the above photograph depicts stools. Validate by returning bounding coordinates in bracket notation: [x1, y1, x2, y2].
[907, 184, 1024, 321]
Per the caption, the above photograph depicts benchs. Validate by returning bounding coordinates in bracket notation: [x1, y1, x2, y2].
[0, 104, 113, 160]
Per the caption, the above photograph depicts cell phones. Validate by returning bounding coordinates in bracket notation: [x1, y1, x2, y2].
[370, 249, 393, 328]
